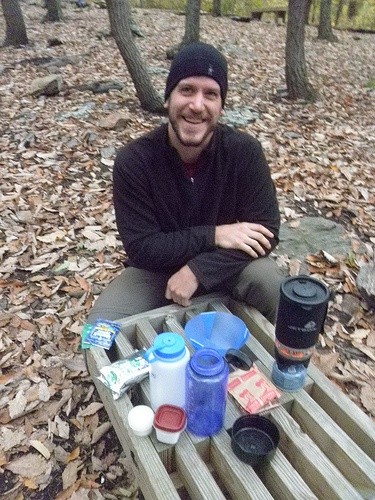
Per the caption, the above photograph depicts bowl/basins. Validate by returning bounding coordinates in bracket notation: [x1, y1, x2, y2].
[185, 311, 250, 356]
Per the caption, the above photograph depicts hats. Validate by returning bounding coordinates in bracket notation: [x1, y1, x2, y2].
[165, 42, 228, 109]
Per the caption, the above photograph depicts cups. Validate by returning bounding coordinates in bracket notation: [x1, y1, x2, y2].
[152, 404, 187, 446]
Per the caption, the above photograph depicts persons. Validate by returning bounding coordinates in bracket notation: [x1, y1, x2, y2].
[85, 44, 290, 387]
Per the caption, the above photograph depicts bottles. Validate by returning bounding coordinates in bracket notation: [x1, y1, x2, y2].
[143, 333, 190, 413]
[184, 349, 229, 435]
[128, 405, 155, 438]
[271, 275, 330, 391]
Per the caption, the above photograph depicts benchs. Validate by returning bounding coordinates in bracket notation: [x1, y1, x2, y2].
[81, 291, 375, 500]
